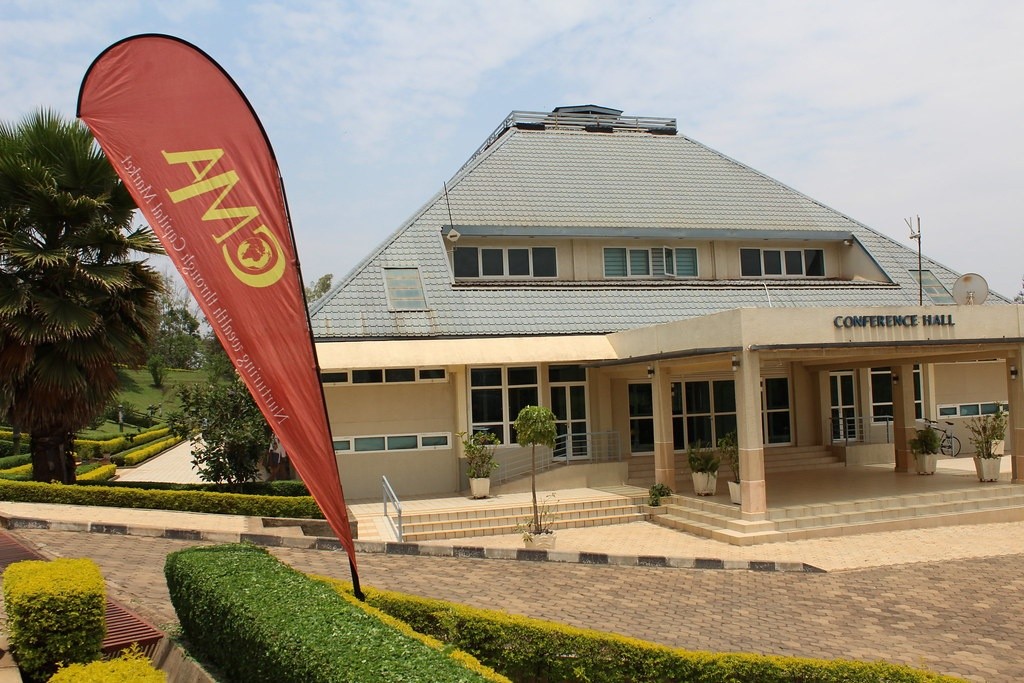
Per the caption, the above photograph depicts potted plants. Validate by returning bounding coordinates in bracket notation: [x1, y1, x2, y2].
[908, 422, 941, 475]
[718, 432, 740, 504]
[686, 439, 721, 496]
[963, 399, 1010, 483]
[454, 429, 500, 499]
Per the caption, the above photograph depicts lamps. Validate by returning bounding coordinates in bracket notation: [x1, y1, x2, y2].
[671, 384, 675, 396]
[647, 364, 655, 379]
[1010, 365, 1018, 380]
[760, 376, 764, 391]
[892, 373, 898, 384]
[732, 355, 740, 371]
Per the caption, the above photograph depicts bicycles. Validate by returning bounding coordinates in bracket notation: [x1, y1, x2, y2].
[917, 418, 962, 457]
